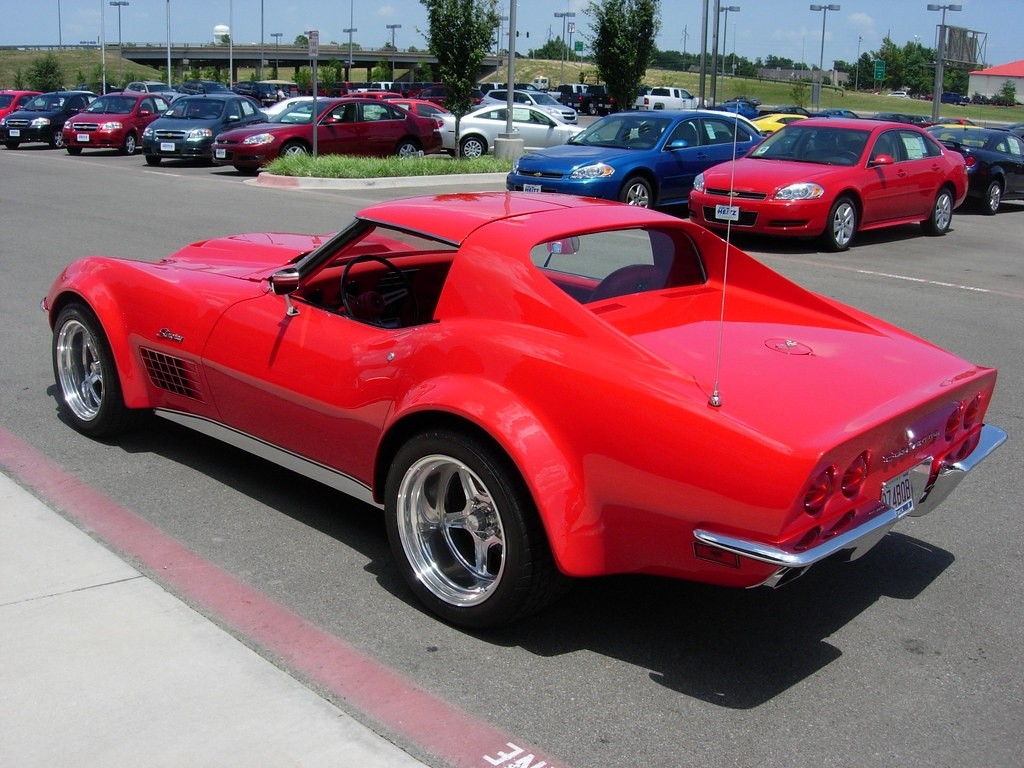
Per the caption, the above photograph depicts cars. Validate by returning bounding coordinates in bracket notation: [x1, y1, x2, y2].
[41, 189, 1009, 632]
[62, 93, 182, 157]
[503, 109, 769, 210]
[141, 94, 268, 168]
[210, 96, 443, 175]
[433, 103, 590, 161]
[687, 117, 970, 252]
[0, 75, 359, 150]
[360, 72, 1024, 218]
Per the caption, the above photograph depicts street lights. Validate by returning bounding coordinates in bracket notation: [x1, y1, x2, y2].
[386, 24, 402, 82]
[270, 32, 283, 79]
[718, 6, 741, 106]
[496, 16, 508, 77]
[927, 4, 964, 123]
[553, 12, 576, 82]
[808, 4, 841, 110]
[303, 31, 312, 73]
[343, 28, 357, 81]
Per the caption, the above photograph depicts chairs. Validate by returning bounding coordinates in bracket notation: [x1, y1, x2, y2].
[636, 123, 697, 146]
[713, 131, 731, 143]
[141, 103, 151, 112]
[49, 98, 60, 108]
[807, 133, 890, 162]
[588, 265, 668, 304]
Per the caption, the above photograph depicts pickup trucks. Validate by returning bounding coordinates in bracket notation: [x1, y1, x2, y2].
[633, 87, 707, 111]
